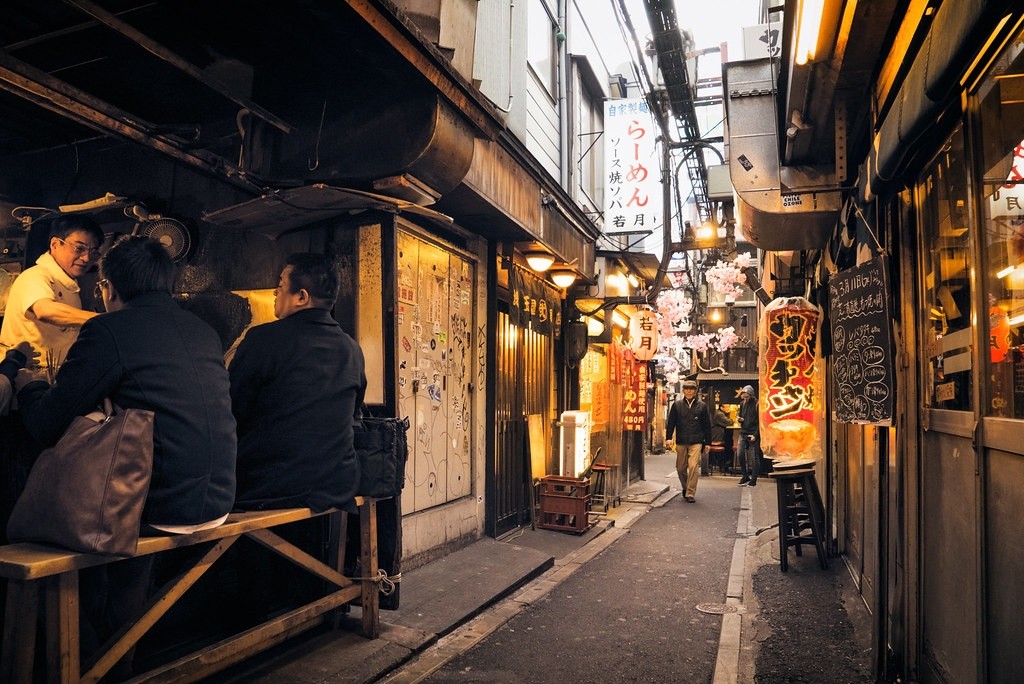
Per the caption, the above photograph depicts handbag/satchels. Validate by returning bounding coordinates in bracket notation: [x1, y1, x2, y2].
[6, 398, 158, 556]
[352, 402, 410, 497]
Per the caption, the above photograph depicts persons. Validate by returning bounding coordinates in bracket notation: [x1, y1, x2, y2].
[737, 385, 762, 487]
[711, 403, 733, 472]
[227, 252, 369, 633]
[0, 208, 110, 373]
[14, 233, 238, 672]
[0, 341, 44, 413]
[665, 380, 713, 504]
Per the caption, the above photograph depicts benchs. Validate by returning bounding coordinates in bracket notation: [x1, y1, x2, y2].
[0, 490, 395, 684]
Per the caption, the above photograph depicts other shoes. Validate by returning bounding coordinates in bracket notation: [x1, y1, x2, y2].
[720, 467, 730, 473]
[740, 477, 751, 483]
[748, 479, 756, 486]
[683, 490, 686, 498]
[686, 497, 695, 503]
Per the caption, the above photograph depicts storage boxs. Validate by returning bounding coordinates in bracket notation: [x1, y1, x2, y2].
[539, 474, 592, 534]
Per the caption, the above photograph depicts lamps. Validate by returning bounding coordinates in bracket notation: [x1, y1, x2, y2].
[525, 253, 555, 272]
[550, 271, 577, 288]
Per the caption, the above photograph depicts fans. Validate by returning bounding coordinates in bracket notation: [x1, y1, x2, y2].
[125, 205, 191, 264]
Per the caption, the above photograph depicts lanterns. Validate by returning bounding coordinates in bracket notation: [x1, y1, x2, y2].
[756, 294, 827, 462]
[628, 310, 659, 362]
[986, 304, 1011, 362]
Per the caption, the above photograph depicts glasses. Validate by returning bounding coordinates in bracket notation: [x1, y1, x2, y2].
[58, 237, 101, 261]
[96, 279, 107, 292]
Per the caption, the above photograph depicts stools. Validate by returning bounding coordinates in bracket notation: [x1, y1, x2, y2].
[766, 456, 828, 571]
[587, 461, 620, 512]
[710, 445, 738, 477]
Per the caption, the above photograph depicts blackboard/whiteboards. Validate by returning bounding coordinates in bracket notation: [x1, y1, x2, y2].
[829, 256, 897, 426]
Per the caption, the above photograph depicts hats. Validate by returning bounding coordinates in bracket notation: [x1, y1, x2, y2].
[683, 380, 697, 388]
[741, 385, 754, 394]
